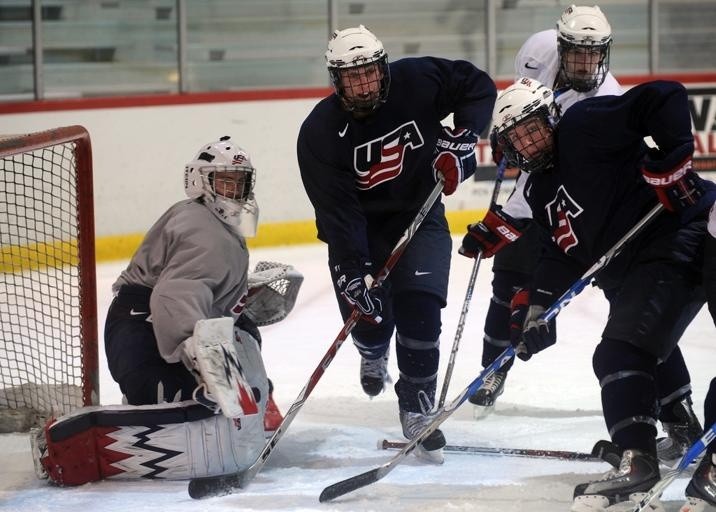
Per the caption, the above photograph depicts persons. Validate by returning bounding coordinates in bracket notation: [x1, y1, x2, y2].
[684, 203, 716, 508]
[493, 77, 715, 504]
[466, 5, 625, 406]
[296, 27, 496, 452]
[30, 138, 307, 492]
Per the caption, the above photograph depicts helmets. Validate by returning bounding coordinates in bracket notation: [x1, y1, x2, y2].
[184, 136, 258, 225]
[325, 25, 391, 114]
[555, 4, 613, 93]
[493, 78, 563, 174]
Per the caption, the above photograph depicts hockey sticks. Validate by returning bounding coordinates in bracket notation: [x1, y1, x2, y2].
[189, 182, 447, 497]
[321, 203, 663, 502]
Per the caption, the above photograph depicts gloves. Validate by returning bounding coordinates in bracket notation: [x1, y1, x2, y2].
[457, 210, 524, 258]
[508, 287, 557, 360]
[332, 255, 393, 325]
[431, 127, 480, 195]
[642, 160, 707, 218]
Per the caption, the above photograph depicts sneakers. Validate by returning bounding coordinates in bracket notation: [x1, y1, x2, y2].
[468, 372, 507, 406]
[574, 407, 714, 506]
[30, 428, 50, 480]
[400, 407, 446, 452]
[360, 347, 393, 396]
